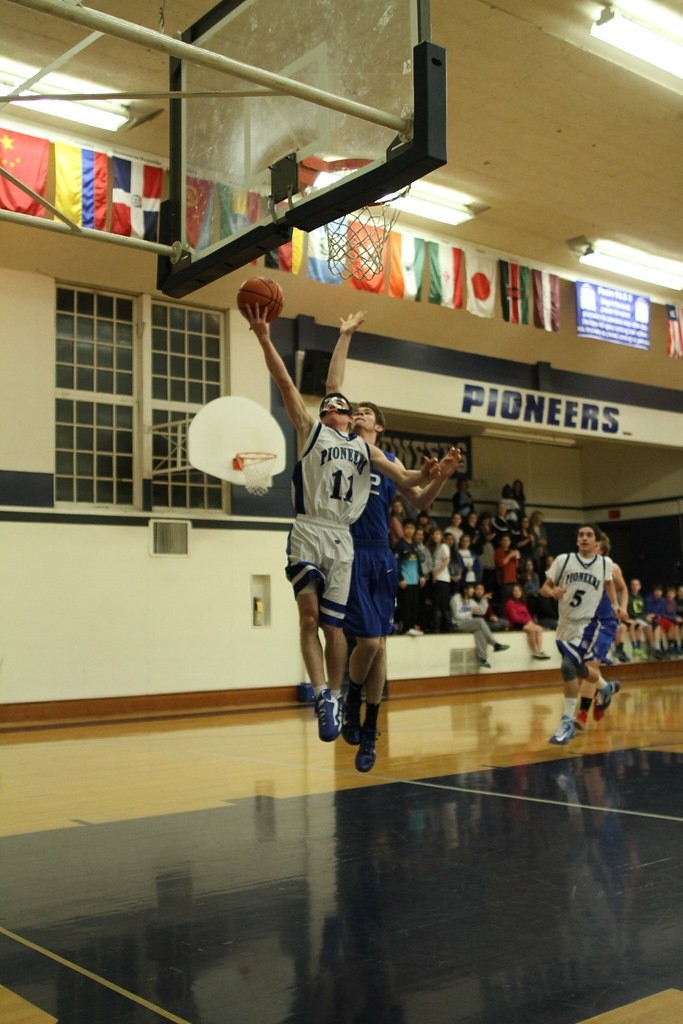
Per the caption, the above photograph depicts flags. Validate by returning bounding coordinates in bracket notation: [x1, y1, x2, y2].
[532, 270, 560, 332]
[167, 174, 303, 274]
[666, 305, 683, 359]
[500, 260, 530, 323]
[466, 251, 497, 319]
[0, 128, 50, 218]
[111, 155, 163, 243]
[576, 281, 651, 350]
[54, 142, 108, 234]
[307, 215, 426, 302]
[428, 240, 463, 309]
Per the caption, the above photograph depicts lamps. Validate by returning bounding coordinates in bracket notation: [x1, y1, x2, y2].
[0, 70, 164, 132]
[303, 169, 490, 226]
[477, 428, 586, 449]
[567, 235, 683, 292]
[590, 7, 683, 77]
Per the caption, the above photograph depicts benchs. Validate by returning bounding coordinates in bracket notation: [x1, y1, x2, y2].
[388, 630, 683, 680]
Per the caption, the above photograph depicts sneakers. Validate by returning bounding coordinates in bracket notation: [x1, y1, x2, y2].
[355, 722, 381, 772]
[594, 698, 604, 722]
[550, 715, 574, 746]
[574, 710, 586, 731]
[315, 689, 344, 742]
[339, 693, 362, 744]
[596, 682, 620, 710]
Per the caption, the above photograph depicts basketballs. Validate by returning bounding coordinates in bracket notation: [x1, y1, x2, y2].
[237, 276, 285, 323]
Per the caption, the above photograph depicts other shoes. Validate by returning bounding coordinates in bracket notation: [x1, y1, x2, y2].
[407, 629, 424, 636]
[478, 658, 491, 669]
[533, 651, 550, 659]
[494, 643, 508, 652]
[602, 645, 683, 664]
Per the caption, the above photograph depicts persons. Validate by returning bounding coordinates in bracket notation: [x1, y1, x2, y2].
[247, 301, 465, 771]
[605, 579, 683, 662]
[390, 479, 560, 666]
[540, 522, 629, 744]
